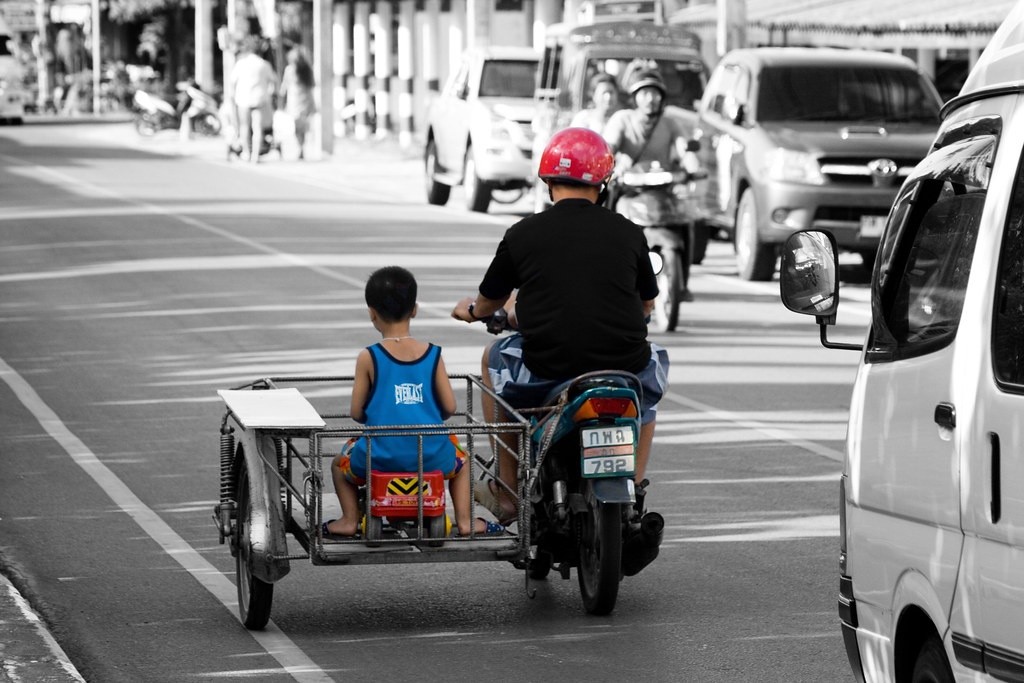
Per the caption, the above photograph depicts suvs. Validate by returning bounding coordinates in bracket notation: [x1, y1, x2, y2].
[423, 46, 541, 212]
[697, 48, 944, 281]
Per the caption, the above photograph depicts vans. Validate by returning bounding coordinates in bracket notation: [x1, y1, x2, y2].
[780, 0, 1024, 683]
[531, 22, 711, 265]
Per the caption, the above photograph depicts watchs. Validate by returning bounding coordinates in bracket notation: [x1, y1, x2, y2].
[468, 299, 482, 320]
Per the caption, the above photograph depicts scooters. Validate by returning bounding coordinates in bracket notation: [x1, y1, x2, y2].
[131, 77, 222, 136]
[601, 139, 709, 329]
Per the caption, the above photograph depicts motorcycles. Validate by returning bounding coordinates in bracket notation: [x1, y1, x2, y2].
[212, 252, 664, 630]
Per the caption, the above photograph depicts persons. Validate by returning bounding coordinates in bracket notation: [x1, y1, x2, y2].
[566, 68, 709, 302]
[311, 265, 504, 539]
[28, 19, 315, 161]
[452, 126, 669, 523]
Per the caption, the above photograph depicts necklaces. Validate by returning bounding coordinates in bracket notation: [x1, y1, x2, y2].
[381, 336, 414, 342]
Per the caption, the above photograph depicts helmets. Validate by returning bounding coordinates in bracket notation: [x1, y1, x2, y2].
[537, 127, 616, 186]
[628, 68, 667, 93]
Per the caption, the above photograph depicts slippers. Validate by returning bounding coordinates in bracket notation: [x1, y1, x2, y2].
[456, 516, 505, 537]
[472, 478, 517, 521]
[313, 519, 361, 540]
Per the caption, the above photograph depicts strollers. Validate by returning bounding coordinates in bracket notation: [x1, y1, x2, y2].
[226, 93, 278, 162]
